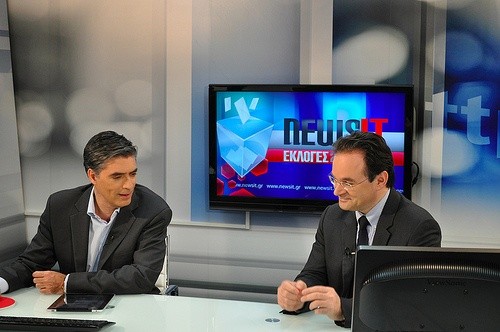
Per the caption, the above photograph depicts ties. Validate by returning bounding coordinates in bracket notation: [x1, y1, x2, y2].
[356, 216, 371, 246]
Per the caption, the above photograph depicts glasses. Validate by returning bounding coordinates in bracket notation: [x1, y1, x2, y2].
[328, 170, 374, 191]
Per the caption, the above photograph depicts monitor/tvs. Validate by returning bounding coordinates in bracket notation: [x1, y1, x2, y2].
[209, 83, 416, 216]
[351, 245, 500, 332]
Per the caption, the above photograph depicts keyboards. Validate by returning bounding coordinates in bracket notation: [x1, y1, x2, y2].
[0, 316, 117, 332]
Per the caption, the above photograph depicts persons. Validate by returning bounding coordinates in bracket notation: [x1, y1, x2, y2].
[277, 132, 442, 328]
[0, 131, 172, 294]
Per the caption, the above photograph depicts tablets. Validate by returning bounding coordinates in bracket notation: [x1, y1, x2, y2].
[47, 292, 116, 312]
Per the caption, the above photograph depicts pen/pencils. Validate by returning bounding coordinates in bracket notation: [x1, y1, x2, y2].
[51, 309, 104, 313]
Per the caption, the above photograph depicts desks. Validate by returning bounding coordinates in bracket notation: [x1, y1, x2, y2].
[0, 287, 351, 332]
